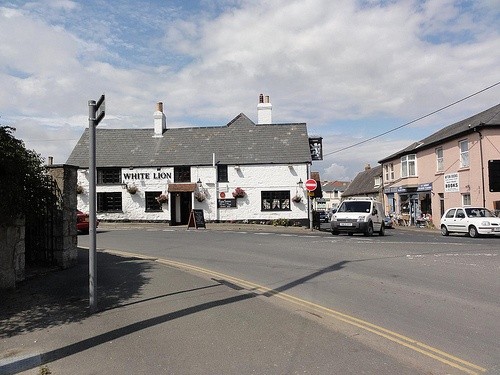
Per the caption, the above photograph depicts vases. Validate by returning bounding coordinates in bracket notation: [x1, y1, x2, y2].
[159, 199, 167, 203]
[294, 199, 300, 203]
[128, 191, 136, 195]
[235, 194, 244, 198]
[197, 198, 204, 203]
[77, 190, 82, 194]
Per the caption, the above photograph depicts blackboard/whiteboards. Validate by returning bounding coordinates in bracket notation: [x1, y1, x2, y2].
[217, 199, 236, 208]
[188, 209, 205, 227]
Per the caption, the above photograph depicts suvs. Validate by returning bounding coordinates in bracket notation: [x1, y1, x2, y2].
[328, 197, 387, 238]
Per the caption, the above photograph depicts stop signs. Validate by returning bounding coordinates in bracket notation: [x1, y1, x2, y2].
[305, 179, 318, 191]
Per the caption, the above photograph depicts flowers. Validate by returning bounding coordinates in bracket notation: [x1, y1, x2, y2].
[291, 196, 303, 202]
[77, 185, 83, 191]
[155, 195, 167, 200]
[232, 188, 245, 197]
[126, 181, 137, 191]
[195, 192, 206, 199]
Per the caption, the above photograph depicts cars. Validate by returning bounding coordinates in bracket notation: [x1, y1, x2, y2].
[318, 211, 329, 223]
[384, 218, 393, 227]
[55, 205, 100, 231]
[440, 205, 500, 238]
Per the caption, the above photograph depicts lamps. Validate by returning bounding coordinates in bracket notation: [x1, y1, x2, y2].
[158, 166, 162, 171]
[288, 165, 293, 169]
[297, 178, 304, 188]
[197, 178, 202, 188]
[129, 167, 134, 170]
[235, 165, 240, 170]
